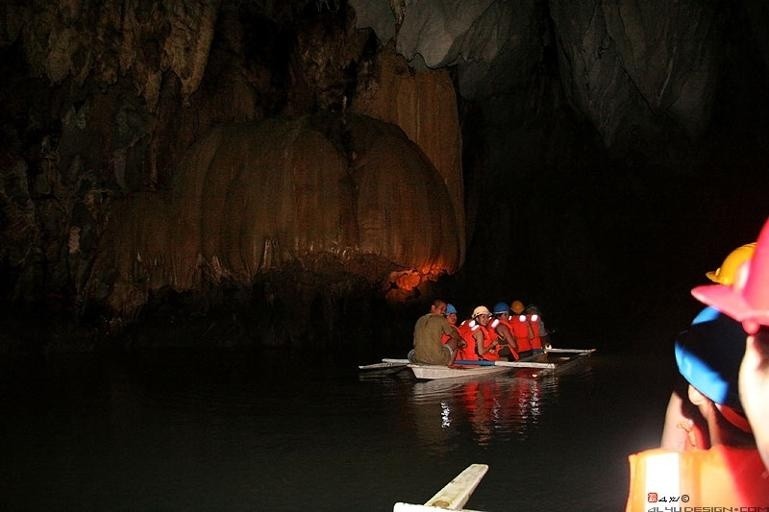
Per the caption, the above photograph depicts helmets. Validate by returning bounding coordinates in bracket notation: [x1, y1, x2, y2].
[472, 306, 490, 318]
[444, 304, 457, 315]
[526, 304, 540, 314]
[691, 218, 769, 334]
[705, 242, 757, 286]
[511, 301, 525, 313]
[493, 303, 509, 314]
[674, 307, 748, 408]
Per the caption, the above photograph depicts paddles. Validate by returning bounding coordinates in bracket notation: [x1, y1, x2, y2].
[383, 358, 556, 369]
[532, 348, 595, 352]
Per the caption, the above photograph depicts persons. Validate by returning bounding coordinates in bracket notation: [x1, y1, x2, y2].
[626, 217, 769, 512]
[407, 302, 547, 368]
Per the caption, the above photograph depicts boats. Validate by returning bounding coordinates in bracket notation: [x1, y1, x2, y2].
[406, 344, 553, 381]
